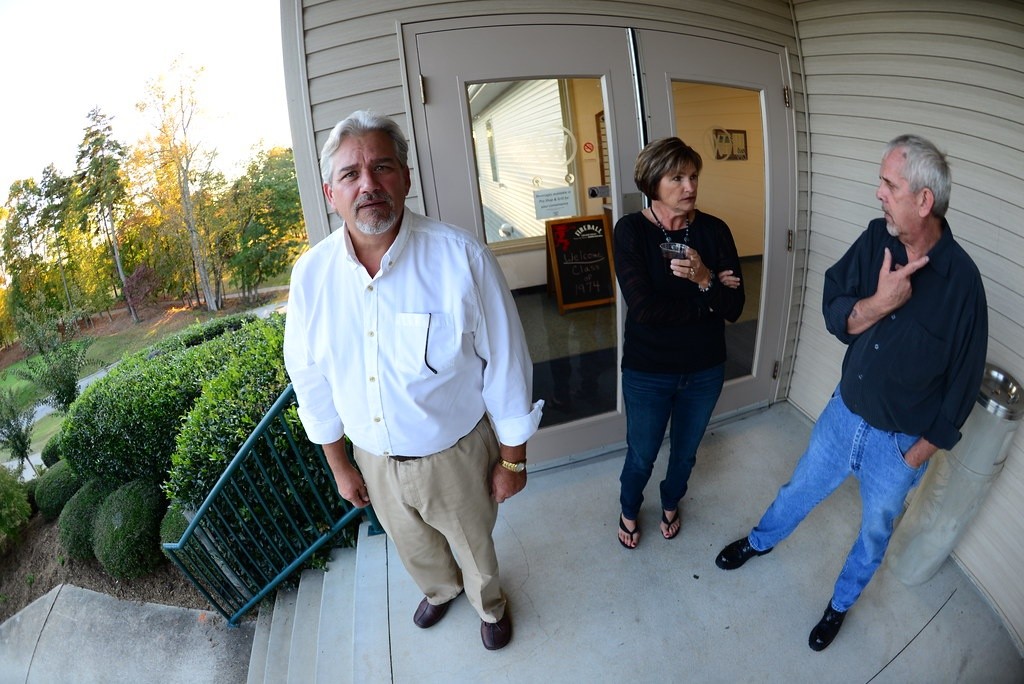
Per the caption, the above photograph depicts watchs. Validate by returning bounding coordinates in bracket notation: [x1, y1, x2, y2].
[500, 458, 527, 473]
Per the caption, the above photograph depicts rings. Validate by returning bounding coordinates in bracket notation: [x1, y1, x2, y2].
[690, 268, 694, 275]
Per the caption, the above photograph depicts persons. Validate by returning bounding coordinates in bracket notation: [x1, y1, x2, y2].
[715, 136, 988, 652]
[611, 138, 746, 548]
[284, 109, 545, 650]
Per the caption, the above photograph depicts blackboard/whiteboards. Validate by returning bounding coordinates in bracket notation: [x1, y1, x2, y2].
[545, 214, 618, 309]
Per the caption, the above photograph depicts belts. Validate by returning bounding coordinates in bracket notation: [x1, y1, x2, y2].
[388, 454, 423, 463]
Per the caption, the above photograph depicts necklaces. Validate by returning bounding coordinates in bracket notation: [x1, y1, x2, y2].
[650, 208, 690, 245]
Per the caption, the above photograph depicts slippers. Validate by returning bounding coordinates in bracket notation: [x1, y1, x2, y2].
[660, 507, 681, 539]
[617, 513, 640, 550]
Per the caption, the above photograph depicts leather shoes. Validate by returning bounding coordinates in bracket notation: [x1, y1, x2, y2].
[715, 536, 773, 571]
[809, 597, 848, 652]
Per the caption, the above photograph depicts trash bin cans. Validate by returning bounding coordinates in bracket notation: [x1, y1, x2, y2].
[883, 361, 1024, 584]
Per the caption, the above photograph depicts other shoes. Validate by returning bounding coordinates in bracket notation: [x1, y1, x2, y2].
[481, 602, 512, 650]
[413, 594, 452, 629]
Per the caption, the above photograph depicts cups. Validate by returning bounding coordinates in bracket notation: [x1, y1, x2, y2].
[660, 243, 689, 285]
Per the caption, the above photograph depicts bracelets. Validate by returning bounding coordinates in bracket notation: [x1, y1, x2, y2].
[699, 270, 712, 292]
